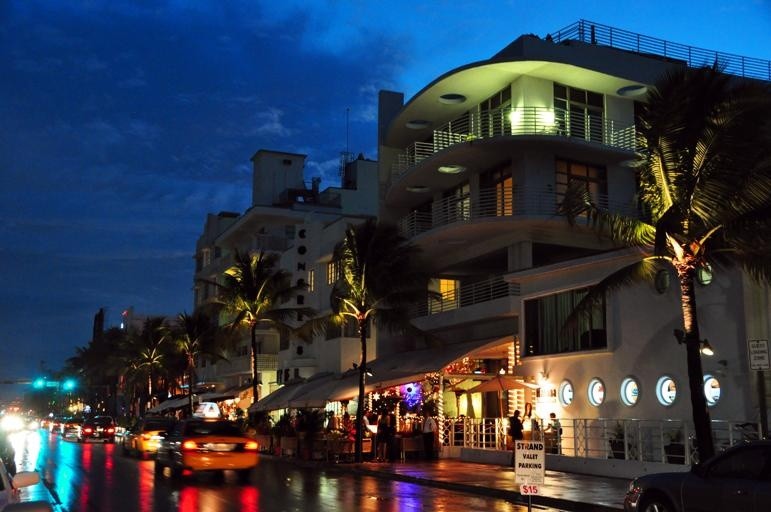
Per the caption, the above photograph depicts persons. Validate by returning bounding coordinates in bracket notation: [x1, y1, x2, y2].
[509, 409, 524, 466]
[422, 409, 438, 461]
[376, 408, 392, 463]
[544, 412, 563, 454]
[325, 410, 340, 429]
[362, 408, 383, 432]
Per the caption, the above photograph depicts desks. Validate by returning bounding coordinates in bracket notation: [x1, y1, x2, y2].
[255, 434, 424, 462]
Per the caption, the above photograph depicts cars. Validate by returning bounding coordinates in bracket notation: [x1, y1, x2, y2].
[122, 401, 261, 477]
[0, 432, 40, 512]
[623, 439, 771, 512]
[21, 415, 115, 443]
